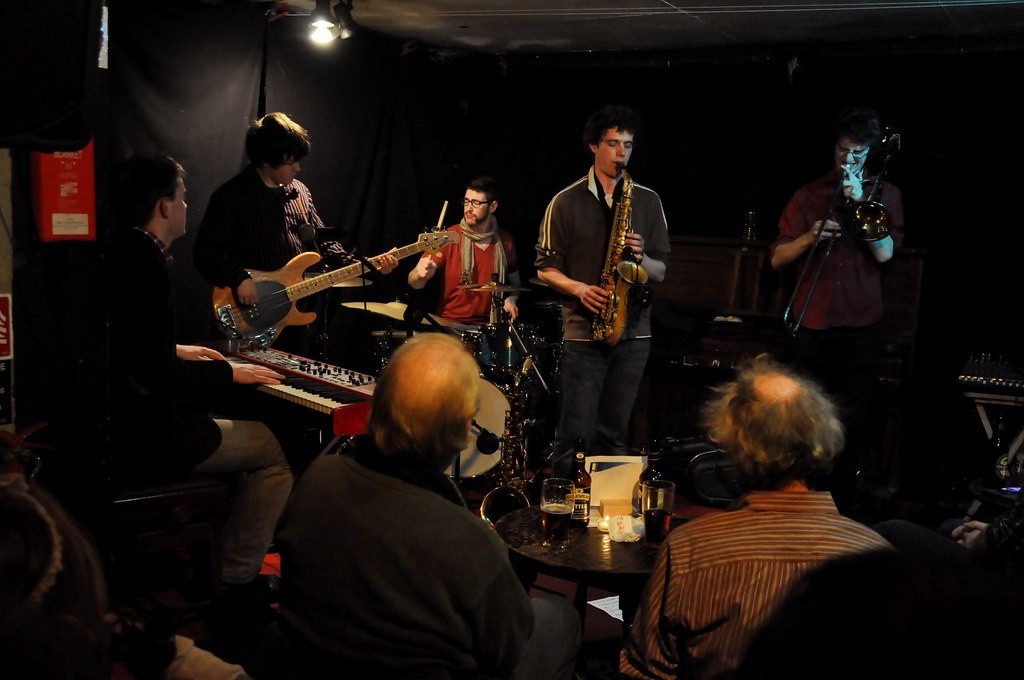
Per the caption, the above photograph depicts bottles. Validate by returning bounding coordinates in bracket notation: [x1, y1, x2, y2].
[569, 436, 591, 528]
[640, 437, 676, 489]
[741, 211, 758, 251]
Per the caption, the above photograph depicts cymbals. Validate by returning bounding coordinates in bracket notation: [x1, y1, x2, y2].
[456, 282, 535, 292]
[341, 301, 455, 327]
[528, 278, 549, 287]
[303, 273, 374, 287]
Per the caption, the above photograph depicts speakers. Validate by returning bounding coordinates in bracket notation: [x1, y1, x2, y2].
[666, 436, 744, 509]
[0, 0, 104, 152]
[961, 390, 1024, 494]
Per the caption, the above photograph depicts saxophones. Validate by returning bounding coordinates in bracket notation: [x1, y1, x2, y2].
[591, 163, 651, 348]
[479, 355, 538, 530]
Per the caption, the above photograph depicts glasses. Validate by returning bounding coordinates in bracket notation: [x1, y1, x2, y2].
[461, 198, 489, 208]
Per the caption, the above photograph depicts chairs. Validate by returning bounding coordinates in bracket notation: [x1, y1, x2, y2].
[65, 315, 235, 625]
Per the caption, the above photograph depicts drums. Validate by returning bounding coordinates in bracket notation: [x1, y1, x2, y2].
[461, 324, 548, 374]
[369, 330, 419, 374]
[442, 373, 513, 479]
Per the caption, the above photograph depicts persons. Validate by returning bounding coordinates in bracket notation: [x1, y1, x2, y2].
[0, 490, 119, 680]
[710, 548, 1024, 680]
[875, 486, 1024, 586]
[407, 176, 523, 325]
[768, 112, 906, 512]
[192, 112, 404, 349]
[619, 355, 900, 679]
[272, 332, 584, 680]
[535, 106, 672, 454]
[107, 152, 295, 598]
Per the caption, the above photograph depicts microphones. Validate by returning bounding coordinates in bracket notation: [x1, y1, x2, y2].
[477, 433, 499, 455]
[623, 245, 634, 257]
[297, 224, 352, 242]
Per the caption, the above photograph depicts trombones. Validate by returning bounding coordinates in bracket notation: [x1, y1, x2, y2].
[783, 125, 901, 339]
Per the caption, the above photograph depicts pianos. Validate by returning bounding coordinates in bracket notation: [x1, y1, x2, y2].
[191, 339, 379, 457]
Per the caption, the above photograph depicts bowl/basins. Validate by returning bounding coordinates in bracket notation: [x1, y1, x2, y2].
[706, 319, 744, 338]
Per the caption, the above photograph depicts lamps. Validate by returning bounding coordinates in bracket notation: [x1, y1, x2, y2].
[307, 0, 356, 50]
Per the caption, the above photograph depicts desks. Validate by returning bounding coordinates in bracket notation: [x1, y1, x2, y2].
[493, 500, 660, 680]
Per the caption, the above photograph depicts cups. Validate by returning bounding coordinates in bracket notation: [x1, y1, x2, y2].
[642, 480, 675, 548]
[540, 479, 575, 554]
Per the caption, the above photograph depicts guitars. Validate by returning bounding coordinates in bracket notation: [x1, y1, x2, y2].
[211, 226, 460, 349]
